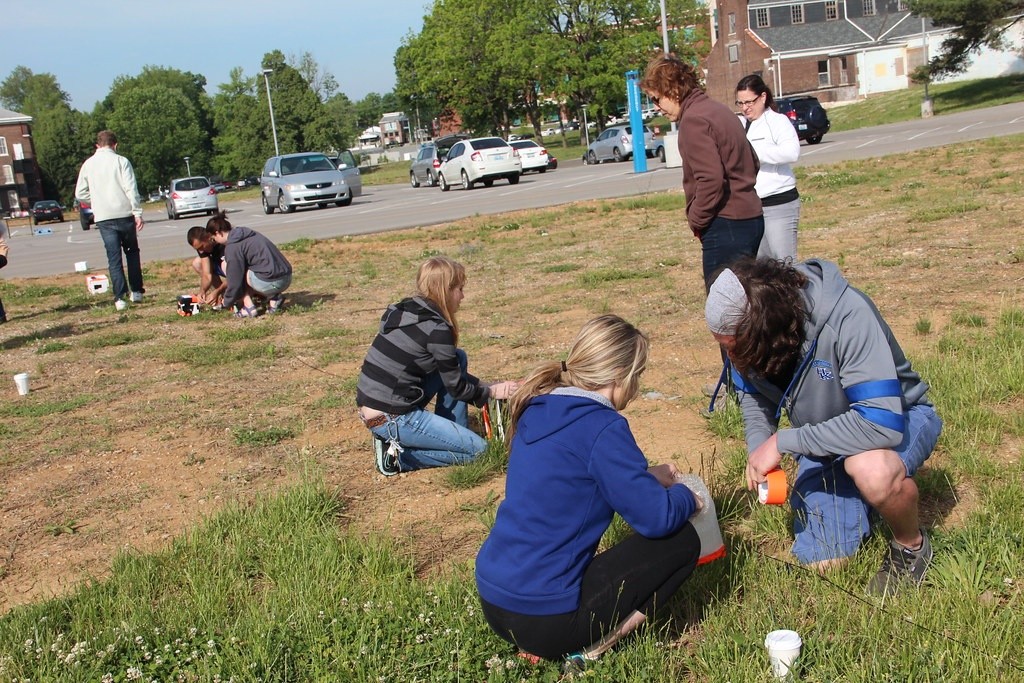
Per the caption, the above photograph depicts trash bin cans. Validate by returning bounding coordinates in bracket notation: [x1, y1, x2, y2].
[921, 97, 935, 118]
[664, 135, 682, 168]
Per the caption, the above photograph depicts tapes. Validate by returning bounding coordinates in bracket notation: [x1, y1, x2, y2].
[757, 468, 786, 505]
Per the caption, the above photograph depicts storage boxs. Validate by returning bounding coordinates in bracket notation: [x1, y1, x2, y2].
[86, 273, 109, 296]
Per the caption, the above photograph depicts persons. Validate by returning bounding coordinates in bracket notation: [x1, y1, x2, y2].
[188, 226, 228, 304]
[639, 56, 766, 417]
[475, 315, 706, 678]
[206, 210, 293, 317]
[75, 131, 145, 310]
[705, 256, 942, 600]
[355, 258, 521, 475]
[733, 75, 801, 267]
[0, 221, 10, 324]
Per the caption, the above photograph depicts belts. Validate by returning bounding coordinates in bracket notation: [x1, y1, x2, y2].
[360, 412, 400, 428]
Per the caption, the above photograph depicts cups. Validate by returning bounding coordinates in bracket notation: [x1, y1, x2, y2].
[764, 629, 802, 683]
[14, 373, 30, 395]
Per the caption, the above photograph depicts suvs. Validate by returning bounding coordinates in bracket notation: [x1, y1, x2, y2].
[767, 94, 831, 145]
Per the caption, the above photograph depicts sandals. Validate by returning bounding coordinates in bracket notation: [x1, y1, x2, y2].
[265, 293, 286, 313]
[233, 306, 259, 318]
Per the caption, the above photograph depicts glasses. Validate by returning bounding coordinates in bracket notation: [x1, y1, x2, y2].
[651, 88, 671, 106]
[736, 95, 762, 108]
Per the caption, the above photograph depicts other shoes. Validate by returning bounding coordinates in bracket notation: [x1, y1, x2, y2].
[566, 653, 611, 680]
[212, 303, 222, 311]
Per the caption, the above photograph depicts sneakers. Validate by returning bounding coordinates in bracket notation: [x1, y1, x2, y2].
[130, 291, 143, 303]
[372, 434, 399, 476]
[114, 299, 127, 311]
[703, 382, 727, 397]
[869, 527, 933, 599]
[702, 393, 729, 418]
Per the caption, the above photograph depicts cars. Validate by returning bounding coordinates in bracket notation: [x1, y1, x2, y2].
[165, 175, 219, 221]
[408, 133, 471, 188]
[78, 201, 95, 231]
[581, 124, 659, 165]
[139, 174, 261, 203]
[507, 107, 663, 176]
[30, 199, 67, 225]
[436, 136, 522, 192]
[259, 149, 363, 215]
[649, 134, 666, 163]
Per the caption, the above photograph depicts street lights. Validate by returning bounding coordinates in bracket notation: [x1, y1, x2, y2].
[258, 68, 280, 156]
[183, 157, 193, 189]
